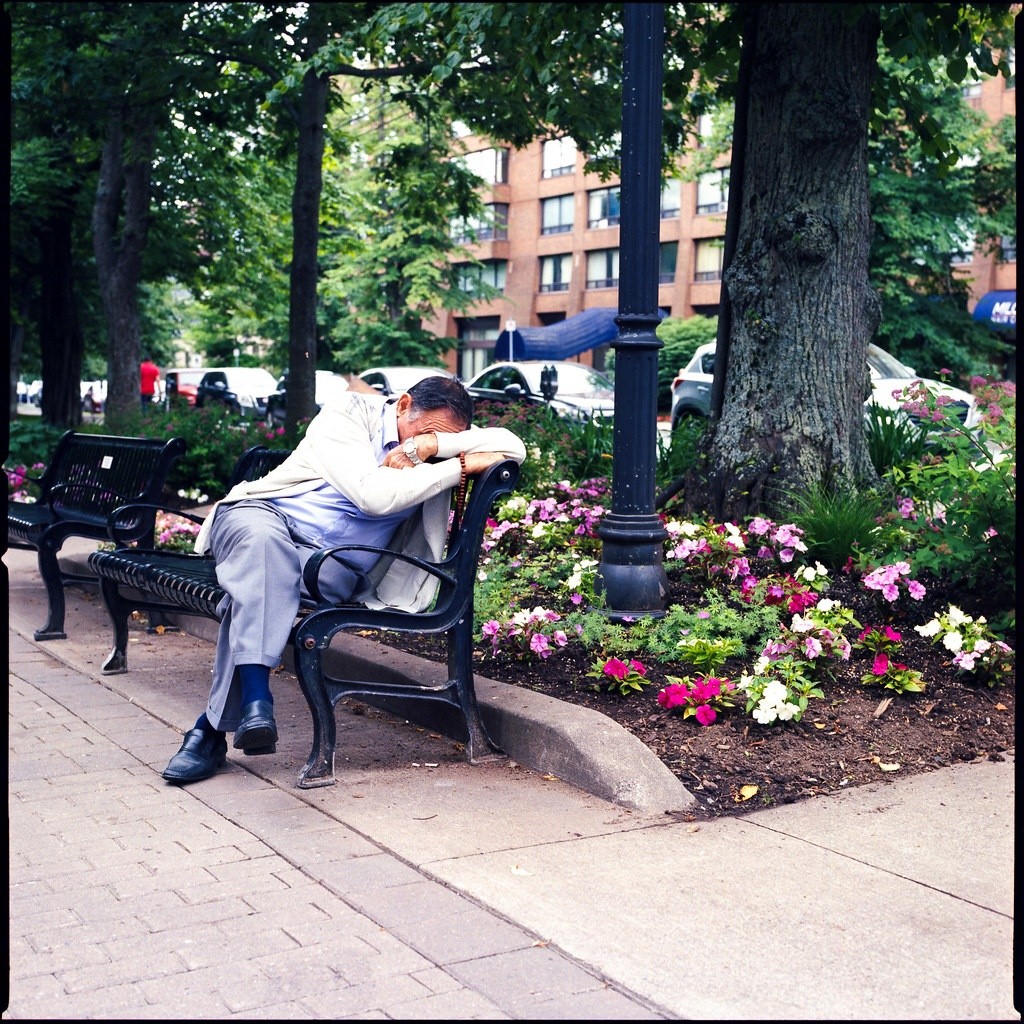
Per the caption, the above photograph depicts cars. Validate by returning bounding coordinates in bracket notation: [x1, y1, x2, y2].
[263, 368, 350, 432]
[163, 369, 207, 411]
[13, 379, 110, 416]
[668, 331, 987, 460]
[193, 367, 280, 420]
[458, 359, 616, 434]
[357, 364, 463, 400]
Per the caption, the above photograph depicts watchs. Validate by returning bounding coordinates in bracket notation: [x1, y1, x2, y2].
[403, 436, 423, 467]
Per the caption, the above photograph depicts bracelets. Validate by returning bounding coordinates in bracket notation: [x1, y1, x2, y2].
[448, 452, 466, 555]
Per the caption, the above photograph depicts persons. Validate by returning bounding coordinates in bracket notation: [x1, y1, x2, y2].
[139, 354, 163, 408]
[163, 378, 527, 786]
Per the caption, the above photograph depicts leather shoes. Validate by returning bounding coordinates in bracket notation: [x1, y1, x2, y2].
[162, 729, 227, 780]
[234, 701, 278, 756]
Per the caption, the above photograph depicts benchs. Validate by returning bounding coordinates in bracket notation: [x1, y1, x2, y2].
[84, 444, 519, 790]
[2, 429, 188, 640]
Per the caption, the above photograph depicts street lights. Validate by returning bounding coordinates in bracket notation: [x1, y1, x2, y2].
[504, 318, 516, 361]
